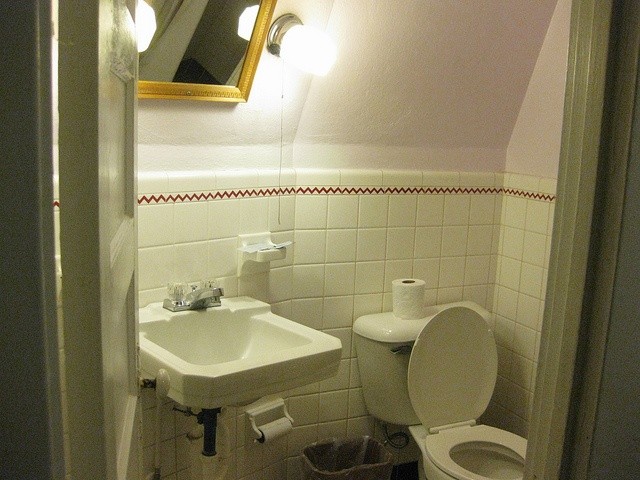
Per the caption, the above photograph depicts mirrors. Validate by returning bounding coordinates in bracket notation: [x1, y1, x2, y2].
[135, 0, 279, 102]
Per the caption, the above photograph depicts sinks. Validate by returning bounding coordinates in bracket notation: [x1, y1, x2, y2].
[139, 296, 343, 409]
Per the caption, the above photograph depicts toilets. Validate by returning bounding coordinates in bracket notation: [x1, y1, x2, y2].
[351, 302, 526, 480]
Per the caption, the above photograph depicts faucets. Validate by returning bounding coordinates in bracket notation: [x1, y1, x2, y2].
[188, 287, 224, 308]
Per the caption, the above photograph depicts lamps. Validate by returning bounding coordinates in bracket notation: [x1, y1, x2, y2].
[236, 5, 261, 40]
[268, 12, 340, 84]
[136, 2, 157, 52]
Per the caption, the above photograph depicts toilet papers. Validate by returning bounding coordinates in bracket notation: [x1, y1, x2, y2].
[255, 417, 295, 444]
[393, 278, 426, 320]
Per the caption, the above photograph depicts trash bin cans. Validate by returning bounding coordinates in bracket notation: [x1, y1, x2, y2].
[301, 434, 393, 480]
